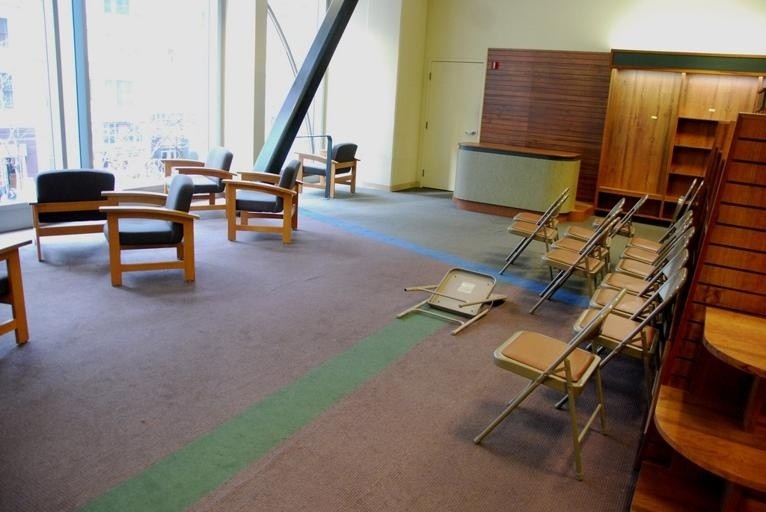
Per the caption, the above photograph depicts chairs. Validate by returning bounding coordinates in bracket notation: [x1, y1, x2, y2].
[159, 144, 237, 220]
[221, 159, 303, 245]
[399, 174, 705, 486]
[28, 170, 118, 267]
[0, 237, 34, 347]
[98, 174, 200, 286]
[295, 142, 361, 198]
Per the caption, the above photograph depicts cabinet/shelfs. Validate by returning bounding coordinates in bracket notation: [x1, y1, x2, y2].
[591, 70, 765, 512]
